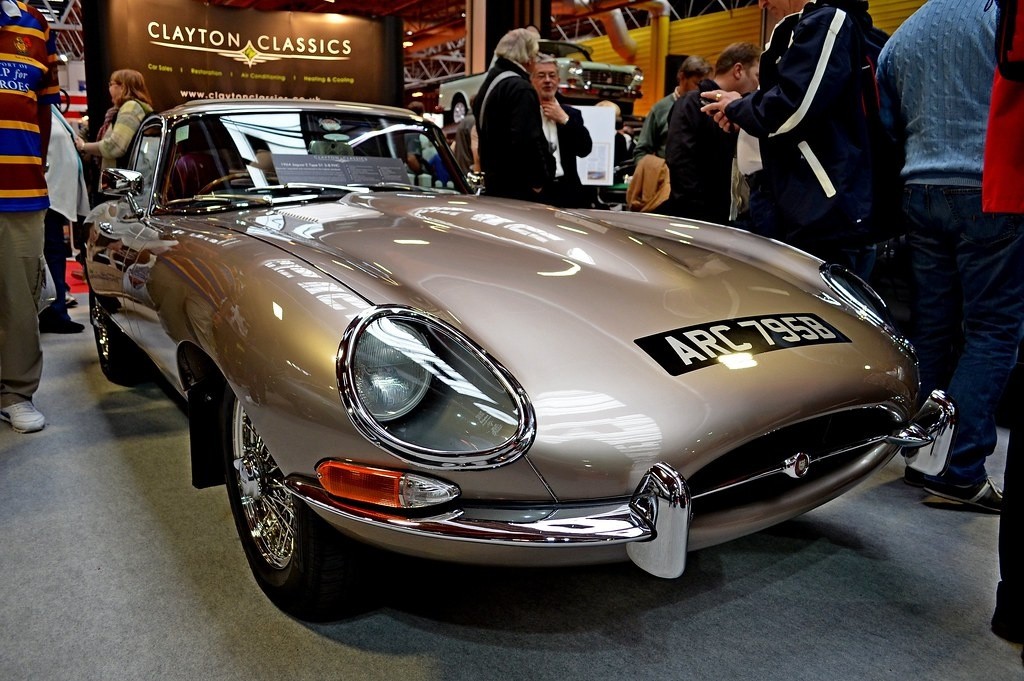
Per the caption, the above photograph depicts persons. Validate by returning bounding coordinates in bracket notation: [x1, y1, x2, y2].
[528, 58, 592, 210]
[0, 0, 61, 435]
[405, 102, 425, 173]
[472, 28, 557, 205]
[451, 94, 480, 178]
[42, 90, 89, 334]
[75, 70, 153, 197]
[590, 0, 1024, 643]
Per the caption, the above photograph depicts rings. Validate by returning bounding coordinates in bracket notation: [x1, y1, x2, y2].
[716, 93, 722, 101]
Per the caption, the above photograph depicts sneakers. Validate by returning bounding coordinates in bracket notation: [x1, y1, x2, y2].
[0, 399, 44, 434]
[905, 467, 1004, 512]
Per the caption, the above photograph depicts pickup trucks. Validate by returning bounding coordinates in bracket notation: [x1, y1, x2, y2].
[435, 39, 644, 123]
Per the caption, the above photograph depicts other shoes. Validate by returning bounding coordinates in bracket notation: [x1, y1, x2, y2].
[39, 318, 85, 334]
[66, 291, 78, 307]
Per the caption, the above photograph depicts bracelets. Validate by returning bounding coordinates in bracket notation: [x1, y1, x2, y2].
[81, 142, 85, 150]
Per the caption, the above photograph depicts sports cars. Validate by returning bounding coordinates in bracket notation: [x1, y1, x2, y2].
[80, 97, 959, 625]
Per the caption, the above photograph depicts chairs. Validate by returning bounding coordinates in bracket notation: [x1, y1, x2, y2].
[168, 152, 227, 201]
